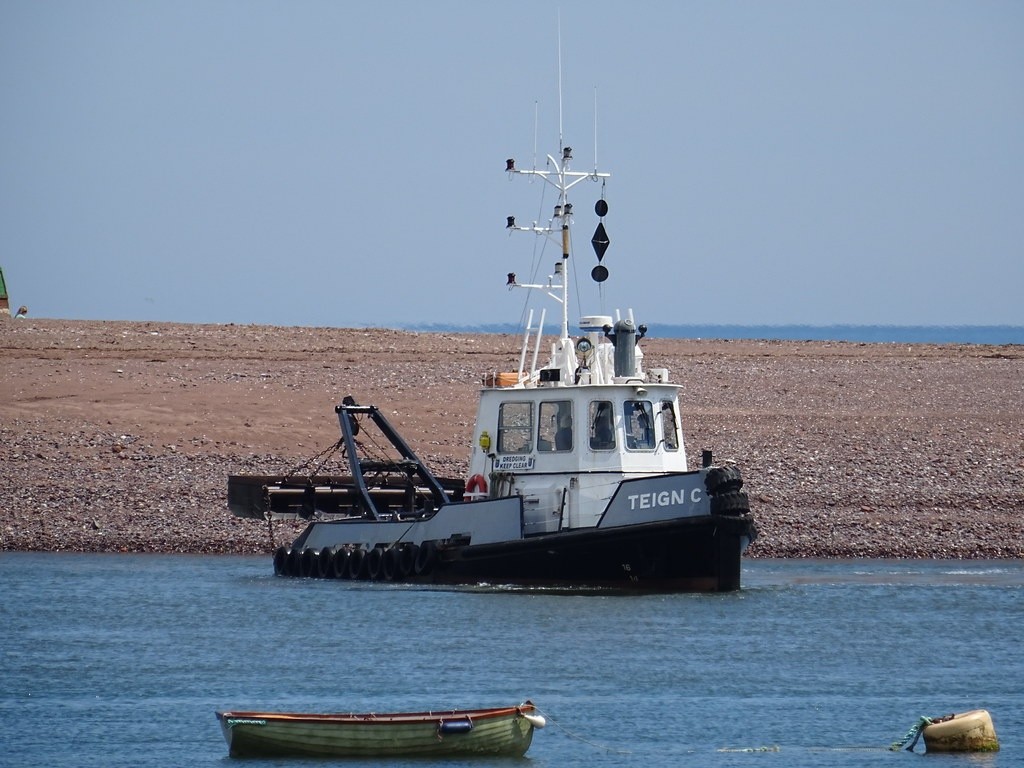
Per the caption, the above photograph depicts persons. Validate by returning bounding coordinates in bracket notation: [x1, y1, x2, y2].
[637, 414, 652, 440]
[593, 416, 612, 442]
[554, 414, 572, 450]
[15, 306, 27, 319]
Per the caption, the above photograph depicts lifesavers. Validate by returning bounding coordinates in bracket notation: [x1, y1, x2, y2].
[276, 542, 437, 581]
[464, 474, 487, 502]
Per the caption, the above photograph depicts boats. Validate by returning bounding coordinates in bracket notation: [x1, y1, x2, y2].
[214, 699, 546, 761]
[219, 12, 757, 599]
